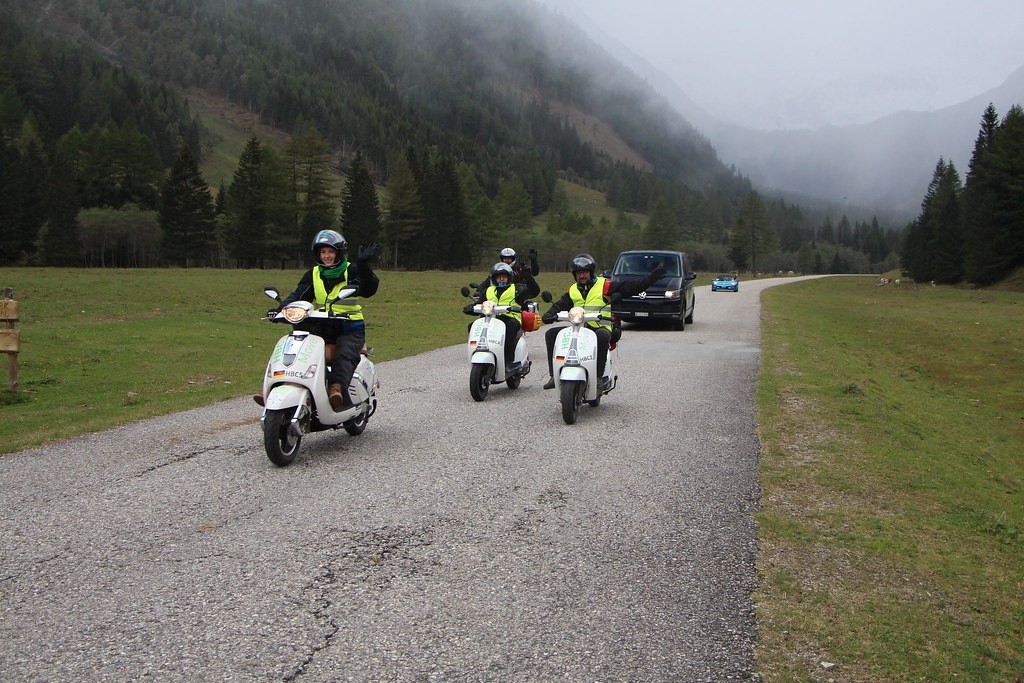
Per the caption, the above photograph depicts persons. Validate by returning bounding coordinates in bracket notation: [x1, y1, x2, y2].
[463, 247, 540, 367]
[734, 274, 737, 280]
[253, 229, 380, 407]
[542, 252, 668, 390]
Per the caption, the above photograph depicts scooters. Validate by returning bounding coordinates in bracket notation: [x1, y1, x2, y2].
[460, 282, 540, 402]
[540, 289, 623, 425]
[258, 285, 381, 468]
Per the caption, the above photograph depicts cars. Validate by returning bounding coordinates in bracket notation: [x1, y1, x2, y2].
[711, 274, 740, 292]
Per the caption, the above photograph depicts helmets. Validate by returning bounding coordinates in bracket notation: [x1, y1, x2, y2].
[491, 262, 514, 284]
[571, 254, 595, 281]
[312, 230, 347, 264]
[500, 248, 516, 263]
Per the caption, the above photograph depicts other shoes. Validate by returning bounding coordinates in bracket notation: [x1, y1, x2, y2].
[329, 383, 343, 409]
[544, 376, 556, 389]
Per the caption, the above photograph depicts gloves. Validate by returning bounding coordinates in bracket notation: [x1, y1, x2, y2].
[648, 266, 668, 281]
[473, 292, 482, 296]
[463, 304, 474, 314]
[542, 312, 554, 323]
[359, 242, 380, 266]
[267, 309, 279, 325]
[519, 262, 531, 278]
[528, 248, 538, 261]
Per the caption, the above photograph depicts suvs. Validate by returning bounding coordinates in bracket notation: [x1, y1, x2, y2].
[603, 250, 697, 331]
